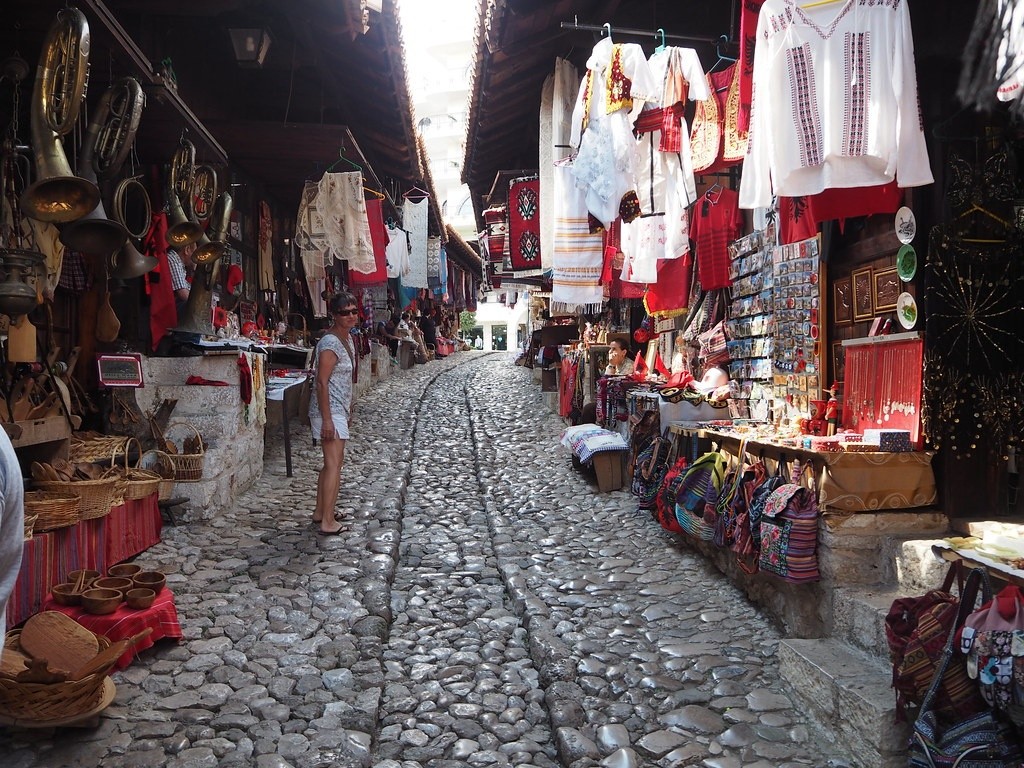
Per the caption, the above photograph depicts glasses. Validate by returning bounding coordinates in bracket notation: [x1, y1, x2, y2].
[334, 309, 358, 315]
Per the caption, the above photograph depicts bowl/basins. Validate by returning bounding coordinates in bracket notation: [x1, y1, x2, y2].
[82, 589, 122, 614]
[131, 571, 166, 594]
[108, 564, 143, 579]
[125, 588, 156, 609]
[67, 569, 103, 587]
[51, 583, 92, 606]
[93, 577, 134, 602]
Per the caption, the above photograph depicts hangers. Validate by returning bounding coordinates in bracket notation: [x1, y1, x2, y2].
[515, 169, 536, 180]
[325, 147, 439, 241]
[591, 24, 738, 93]
[704, 171, 724, 199]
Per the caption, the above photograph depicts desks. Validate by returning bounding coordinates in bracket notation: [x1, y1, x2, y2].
[45, 582, 173, 667]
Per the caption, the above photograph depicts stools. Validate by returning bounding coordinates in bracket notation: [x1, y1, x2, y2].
[564, 424, 623, 491]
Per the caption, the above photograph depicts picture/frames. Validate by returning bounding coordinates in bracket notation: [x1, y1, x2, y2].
[832, 264, 902, 326]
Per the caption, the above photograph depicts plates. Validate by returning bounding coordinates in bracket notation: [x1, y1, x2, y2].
[897, 292, 918, 330]
[896, 243, 916, 282]
[895, 207, 916, 245]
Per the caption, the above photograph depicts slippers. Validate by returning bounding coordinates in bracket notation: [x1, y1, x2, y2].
[312, 510, 347, 522]
[318, 526, 351, 534]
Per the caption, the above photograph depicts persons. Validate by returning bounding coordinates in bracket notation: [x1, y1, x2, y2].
[397, 314, 418, 355]
[167, 242, 197, 312]
[308, 292, 356, 535]
[475, 334, 482, 348]
[376, 315, 400, 363]
[420, 309, 437, 348]
[583, 338, 635, 424]
[0, 424, 24, 656]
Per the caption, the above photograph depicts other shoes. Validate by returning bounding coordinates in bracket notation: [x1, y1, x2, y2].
[389, 355, 393, 361]
[393, 360, 399, 364]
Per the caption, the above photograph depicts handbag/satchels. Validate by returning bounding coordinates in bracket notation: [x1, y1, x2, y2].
[426, 343, 435, 360]
[628, 400, 821, 582]
[884, 559, 1024, 768]
[414, 354, 427, 364]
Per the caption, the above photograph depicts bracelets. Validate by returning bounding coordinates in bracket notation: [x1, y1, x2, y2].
[607, 365, 616, 370]
[415, 326, 417, 329]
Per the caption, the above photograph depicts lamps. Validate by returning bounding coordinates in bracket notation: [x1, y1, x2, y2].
[224, 21, 277, 68]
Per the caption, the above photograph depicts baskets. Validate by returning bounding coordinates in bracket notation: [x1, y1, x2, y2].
[0, 629, 117, 721]
[134, 450, 176, 500]
[30, 465, 116, 520]
[160, 422, 203, 481]
[69, 434, 128, 463]
[115, 438, 163, 497]
[282, 313, 310, 343]
[22, 512, 37, 541]
[24, 492, 81, 531]
[110, 443, 130, 506]
